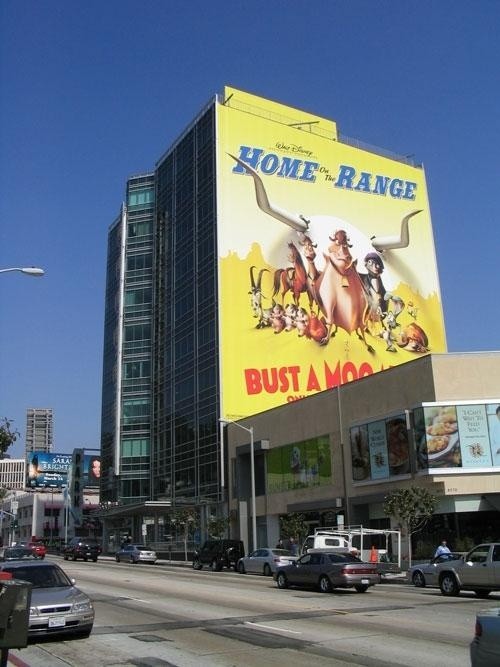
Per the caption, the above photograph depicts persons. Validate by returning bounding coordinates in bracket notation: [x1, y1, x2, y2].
[86, 456, 100, 486]
[434, 541, 454, 561]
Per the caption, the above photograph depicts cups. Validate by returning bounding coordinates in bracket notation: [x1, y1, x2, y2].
[352, 455, 369, 481]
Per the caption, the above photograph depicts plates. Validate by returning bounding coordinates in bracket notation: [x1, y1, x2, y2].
[385, 417, 410, 466]
[417, 413, 459, 460]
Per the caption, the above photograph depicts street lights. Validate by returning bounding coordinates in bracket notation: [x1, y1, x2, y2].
[219, 417, 259, 551]
[1, 267, 46, 282]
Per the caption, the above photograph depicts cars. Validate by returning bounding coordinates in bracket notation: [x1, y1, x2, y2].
[407, 543, 500, 596]
[190, 522, 404, 593]
[468, 605, 500, 667]
[0, 536, 106, 641]
[116, 543, 158, 563]
